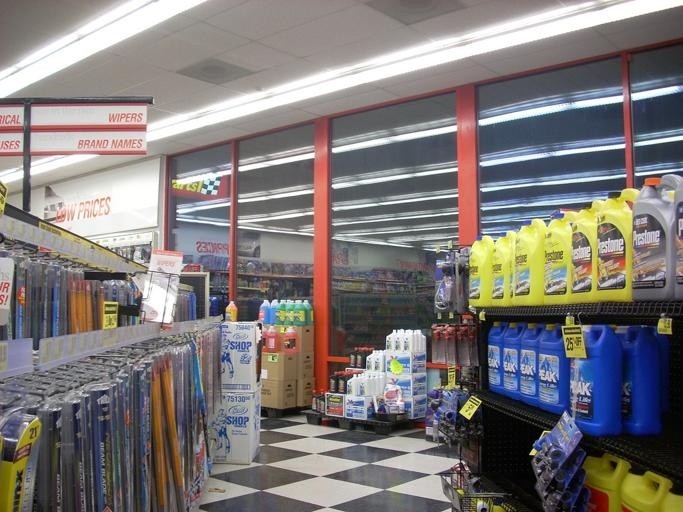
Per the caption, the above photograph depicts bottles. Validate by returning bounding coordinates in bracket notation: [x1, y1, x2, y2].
[311, 329, 427, 414]
[256, 298, 314, 353]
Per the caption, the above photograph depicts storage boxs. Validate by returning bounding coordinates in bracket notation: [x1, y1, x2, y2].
[209, 321, 427, 465]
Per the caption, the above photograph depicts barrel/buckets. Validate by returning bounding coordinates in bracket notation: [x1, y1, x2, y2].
[384, 383, 403, 406]
[612, 325, 663, 435]
[569, 325, 623, 437]
[286, 301, 295, 324]
[270, 299, 279, 324]
[581, 452, 632, 512]
[258, 300, 270, 324]
[295, 299, 308, 326]
[633, 174, 682, 301]
[511, 218, 547, 306]
[674, 175, 683, 300]
[619, 468, 683, 511]
[469, 235, 494, 307]
[569, 200, 604, 303]
[520, 323, 544, 407]
[543, 211, 578, 304]
[277, 300, 289, 324]
[302, 300, 315, 325]
[488, 322, 508, 395]
[503, 322, 525, 401]
[595, 187, 639, 304]
[347, 372, 385, 396]
[366, 349, 385, 371]
[385, 328, 426, 352]
[654, 325, 670, 414]
[490, 230, 516, 306]
[538, 323, 569, 415]
